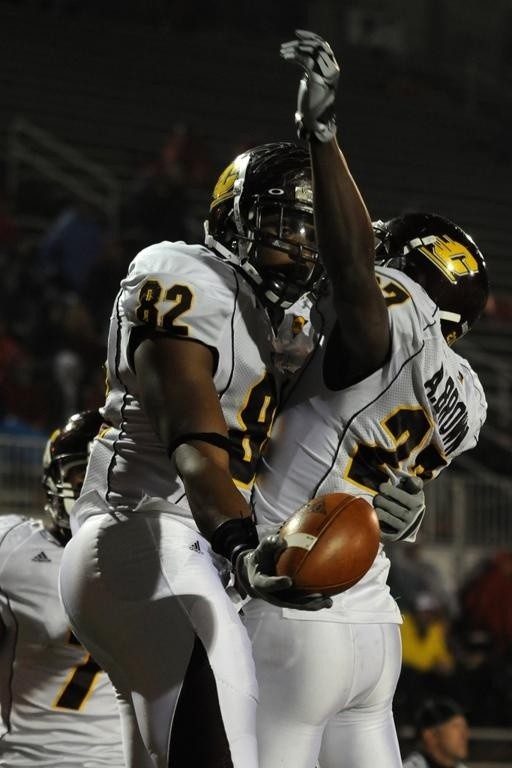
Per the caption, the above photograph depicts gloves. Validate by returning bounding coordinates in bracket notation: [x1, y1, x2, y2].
[372, 476, 428, 546]
[281, 28, 341, 144]
[227, 535, 332, 610]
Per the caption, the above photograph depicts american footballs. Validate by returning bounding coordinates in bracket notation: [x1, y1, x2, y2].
[275, 493, 381, 596]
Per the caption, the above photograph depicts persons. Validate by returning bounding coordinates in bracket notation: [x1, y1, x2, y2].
[381, 538, 497, 768]
[219, 28, 492, 768]
[0, 411, 158, 767]
[55, 138, 331, 766]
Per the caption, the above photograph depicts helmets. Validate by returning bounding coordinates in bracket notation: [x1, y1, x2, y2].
[203, 142, 330, 312]
[38, 409, 101, 542]
[373, 212, 489, 348]
